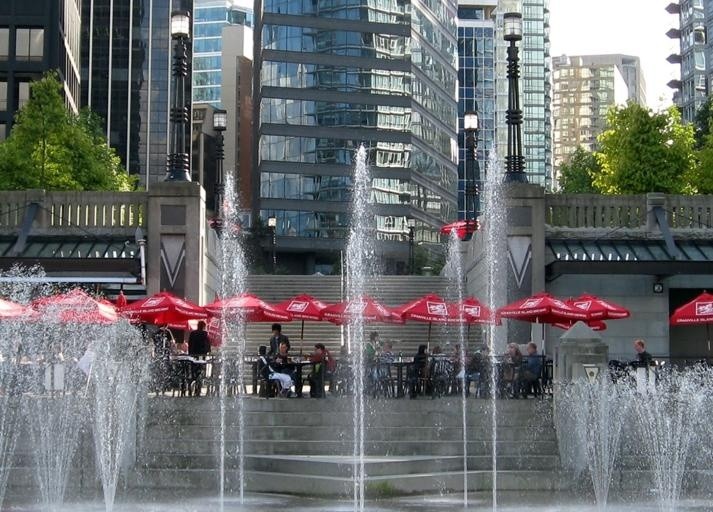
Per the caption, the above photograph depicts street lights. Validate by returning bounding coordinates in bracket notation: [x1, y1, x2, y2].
[212, 109, 228, 238]
[503, 11, 528, 182]
[408, 215, 417, 276]
[164, 9, 192, 181]
[463, 110, 478, 240]
[267, 215, 277, 275]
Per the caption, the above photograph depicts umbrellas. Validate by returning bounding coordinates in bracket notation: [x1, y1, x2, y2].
[671, 289, 713, 366]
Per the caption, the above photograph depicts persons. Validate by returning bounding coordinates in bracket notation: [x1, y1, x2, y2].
[630, 339, 653, 362]
[147, 322, 545, 399]
